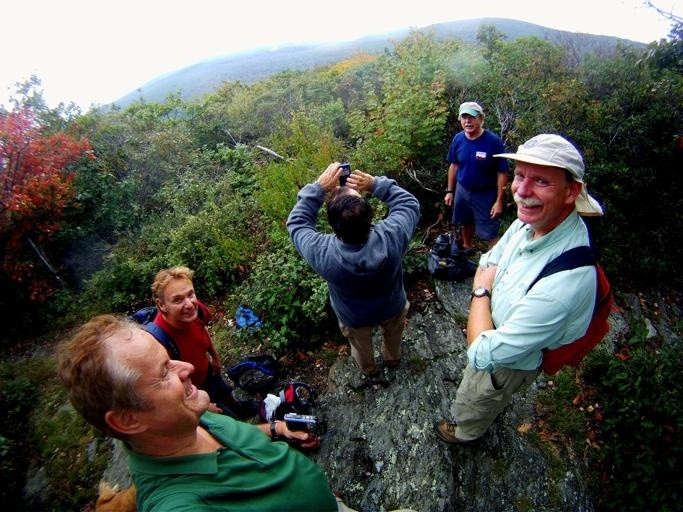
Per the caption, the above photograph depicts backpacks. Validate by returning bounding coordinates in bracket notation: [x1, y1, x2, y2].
[122, 304, 203, 360]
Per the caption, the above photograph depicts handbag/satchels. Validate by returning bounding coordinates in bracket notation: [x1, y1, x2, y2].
[225, 353, 318, 423]
[427, 232, 477, 280]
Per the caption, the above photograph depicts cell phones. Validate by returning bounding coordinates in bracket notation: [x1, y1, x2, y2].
[337, 163, 352, 188]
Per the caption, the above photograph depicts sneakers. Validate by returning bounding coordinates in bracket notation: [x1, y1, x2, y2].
[433, 419, 485, 447]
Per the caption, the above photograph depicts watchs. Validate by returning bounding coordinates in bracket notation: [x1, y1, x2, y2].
[269, 418, 281, 440]
[469, 286, 490, 299]
[445, 188, 454, 193]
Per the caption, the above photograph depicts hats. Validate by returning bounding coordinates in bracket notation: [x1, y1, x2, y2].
[492, 133, 603, 218]
[457, 102, 482, 120]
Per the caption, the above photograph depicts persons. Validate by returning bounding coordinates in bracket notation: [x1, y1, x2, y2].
[143, 264, 263, 421]
[285, 162, 421, 388]
[435, 132, 604, 451]
[443, 101, 509, 252]
[61, 315, 362, 512]
